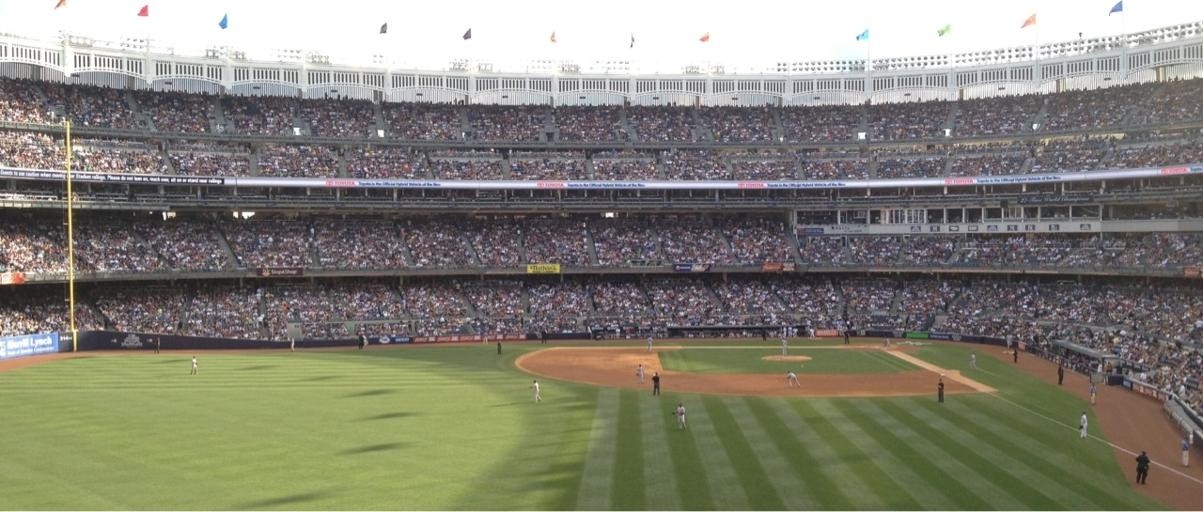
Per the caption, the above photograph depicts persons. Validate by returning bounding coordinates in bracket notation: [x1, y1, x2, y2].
[936, 378, 944, 403]
[532, 379, 542, 403]
[496, 340, 502, 354]
[378, 98, 947, 349]
[671, 402, 687, 430]
[2, 76, 392, 375]
[785, 370, 801, 388]
[1135, 450, 1150, 485]
[1079, 410, 1089, 439]
[636, 364, 661, 396]
[944, 75, 1202, 421]
[1179, 436, 1189, 467]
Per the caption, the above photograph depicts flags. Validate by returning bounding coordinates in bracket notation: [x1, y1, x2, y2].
[54, 0, 65, 10]
[137, 5, 148, 16]
[1021, 13, 1037, 29]
[550, 32, 556, 42]
[856, 29, 869, 41]
[462, 28, 471, 40]
[218, 14, 228, 29]
[937, 24, 950, 37]
[379, 23, 387, 33]
[1108, 1, 1123, 16]
[630, 35, 635, 48]
[700, 33, 709, 42]
